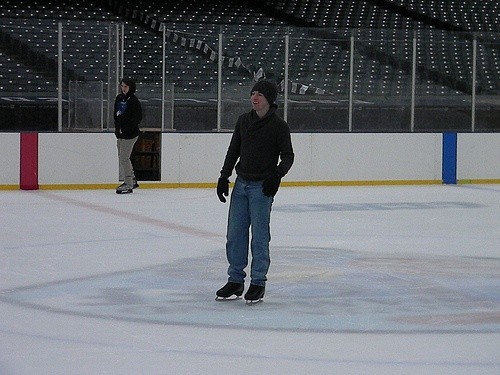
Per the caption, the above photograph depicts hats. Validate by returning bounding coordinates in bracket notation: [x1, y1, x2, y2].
[250, 80, 278, 106]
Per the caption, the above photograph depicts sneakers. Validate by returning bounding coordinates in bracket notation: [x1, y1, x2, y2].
[244, 283, 265, 304]
[215, 281, 245, 301]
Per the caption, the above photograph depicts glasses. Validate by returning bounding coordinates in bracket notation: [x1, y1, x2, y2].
[120, 84, 128, 89]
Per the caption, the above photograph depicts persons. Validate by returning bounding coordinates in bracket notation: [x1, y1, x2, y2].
[216, 79, 294, 300]
[113, 78, 142, 190]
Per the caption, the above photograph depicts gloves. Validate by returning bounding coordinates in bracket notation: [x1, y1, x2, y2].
[261, 176, 281, 198]
[216, 177, 231, 203]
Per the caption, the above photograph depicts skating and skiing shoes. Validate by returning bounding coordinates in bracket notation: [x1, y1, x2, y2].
[115, 182, 133, 193]
[132, 179, 139, 189]
